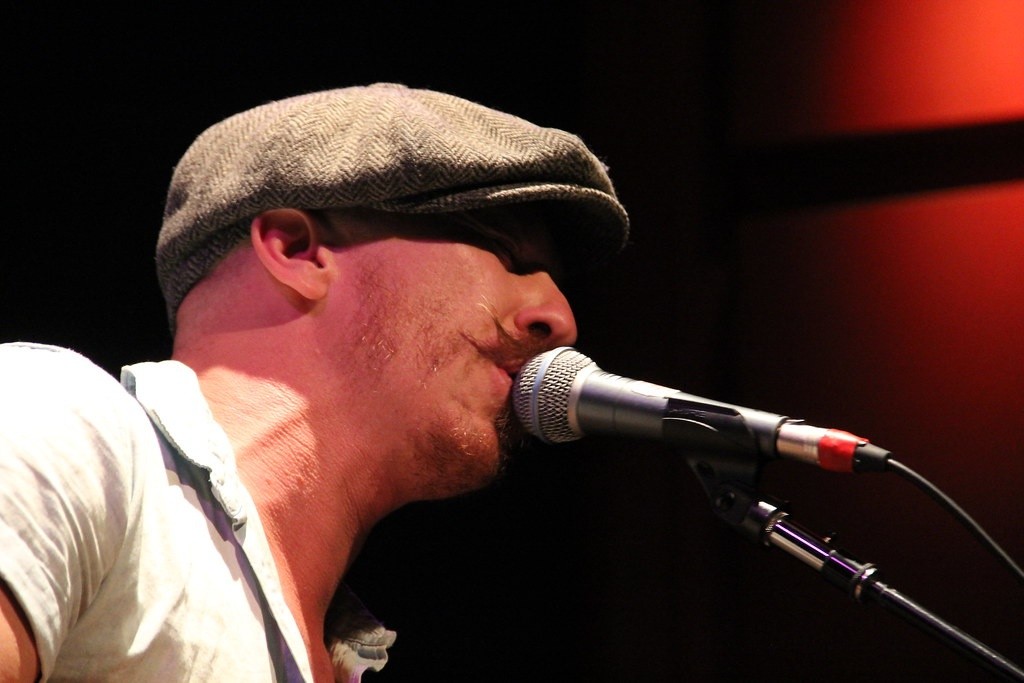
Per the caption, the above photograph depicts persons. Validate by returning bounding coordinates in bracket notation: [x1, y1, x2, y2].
[0, 82, 631, 683]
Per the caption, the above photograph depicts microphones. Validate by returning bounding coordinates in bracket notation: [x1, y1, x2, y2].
[512, 345, 892, 476]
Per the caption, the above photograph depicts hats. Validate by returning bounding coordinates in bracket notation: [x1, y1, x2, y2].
[154, 82, 627, 331]
